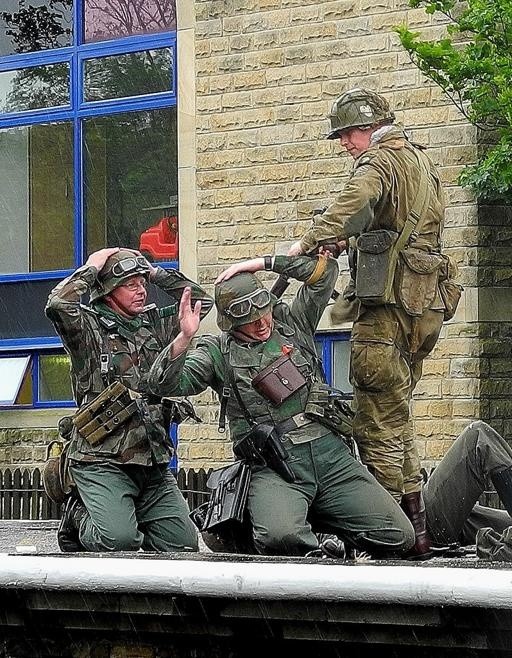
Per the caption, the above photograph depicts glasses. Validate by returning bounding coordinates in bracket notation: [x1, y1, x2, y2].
[118, 281, 147, 289]
[222, 288, 271, 317]
[104, 256, 149, 279]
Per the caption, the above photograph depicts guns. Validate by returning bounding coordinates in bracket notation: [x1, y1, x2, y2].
[270, 241, 340, 298]
[234, 429, 295, 484]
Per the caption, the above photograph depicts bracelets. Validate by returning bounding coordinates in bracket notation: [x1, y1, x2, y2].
[263, 256, 274, 274]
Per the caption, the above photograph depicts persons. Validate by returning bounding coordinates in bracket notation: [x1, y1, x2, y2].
[283, 86, 465, 561]
[43, 245, 215, 554]
[197, 419, 512, 555]
[146, 255, 418, 559]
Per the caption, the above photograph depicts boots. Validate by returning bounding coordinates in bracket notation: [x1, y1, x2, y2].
[372, 491, 434, 560]
[57, 497, 85, 552]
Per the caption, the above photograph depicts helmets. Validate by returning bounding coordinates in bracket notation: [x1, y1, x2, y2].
[215, 273, 275, 331]
[89, 250, 152, 305]
[327, 87, 394, 140]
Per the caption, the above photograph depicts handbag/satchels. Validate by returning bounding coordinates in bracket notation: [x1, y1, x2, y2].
[201, 460, 250, 530]
[355, 230, 399, 302]
